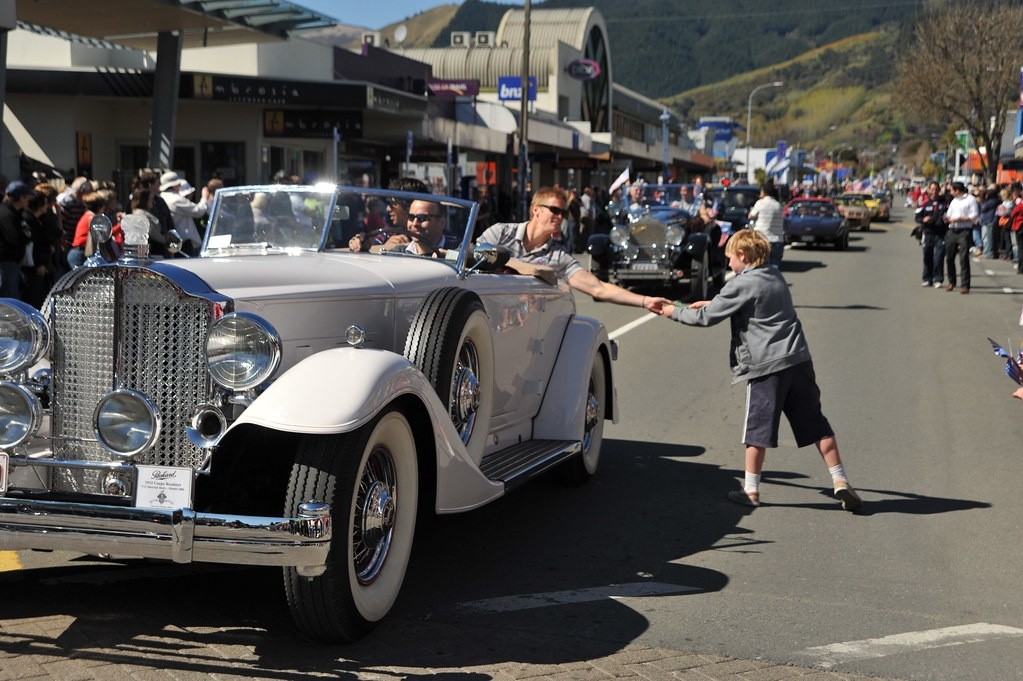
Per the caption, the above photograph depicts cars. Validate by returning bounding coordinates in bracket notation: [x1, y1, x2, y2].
[585, 181, 733, 304]
[699, 181, 891, 252]
[0, 181, 621, 648]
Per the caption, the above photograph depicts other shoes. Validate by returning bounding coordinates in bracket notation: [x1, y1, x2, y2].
[1013, 262, 1019, 269]
[921, 280, 933, 287]
[987, 253, 1000, 259]
[935, 282, 943, 288]
[975, 248, 983, 256]
[946, 283, 955, 291]
[960, 286, 969, 293]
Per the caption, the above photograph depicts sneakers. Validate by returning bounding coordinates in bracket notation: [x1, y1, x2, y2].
[729, 489, 760, 506]
[832, 478, 864, 510]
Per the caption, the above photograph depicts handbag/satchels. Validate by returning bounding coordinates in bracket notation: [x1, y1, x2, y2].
[911, 226, 922, 239]
[999, 216, 1011, 229]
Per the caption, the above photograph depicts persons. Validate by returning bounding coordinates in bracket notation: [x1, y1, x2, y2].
[662, 229, 860, 509]
[454, 185, 603, 251]
[905, 180, 1023, 293]
[748, 183, 783, 262]
[0, 165, 451, 308]
[474, 186, 674, 317]
[605, 172, 856, 215]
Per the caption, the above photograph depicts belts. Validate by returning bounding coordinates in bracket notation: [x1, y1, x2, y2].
[74, 246, 86, 251]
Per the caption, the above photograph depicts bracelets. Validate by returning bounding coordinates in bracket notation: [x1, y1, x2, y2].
[642, 296, 646, 307]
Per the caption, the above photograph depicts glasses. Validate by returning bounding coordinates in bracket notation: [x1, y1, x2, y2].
[386, 199, 400, 209]
[536, 203, 570, 219]
[406, 214, 440, 223]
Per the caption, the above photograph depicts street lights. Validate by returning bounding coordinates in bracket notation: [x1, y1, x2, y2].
[930, 131, 949, 182]
[812, 125, 837, 187]
[829, 141, 854, 184]
[743, 80, 783, 184]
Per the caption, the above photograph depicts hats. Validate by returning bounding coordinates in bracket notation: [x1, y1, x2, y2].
[176, 178, 195, 196]
[5, 181, 32, 198]
[159, 171, 181, 191]
